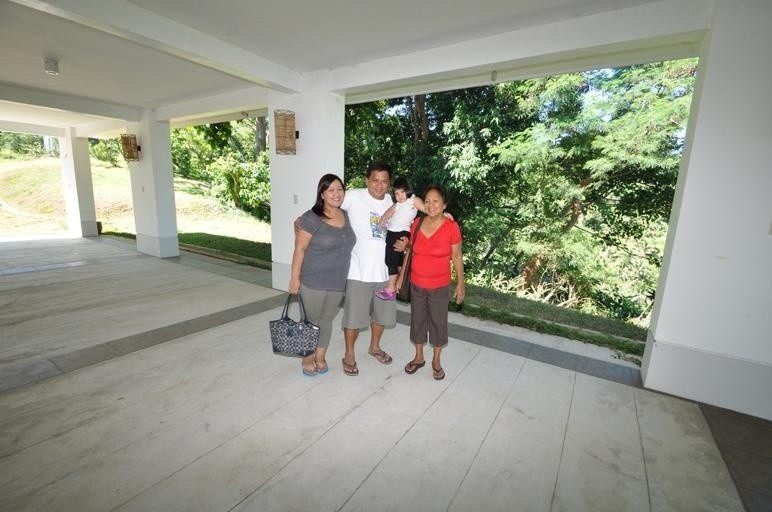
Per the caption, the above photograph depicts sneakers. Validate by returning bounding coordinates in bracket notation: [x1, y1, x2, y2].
[373, 285, 397, 300]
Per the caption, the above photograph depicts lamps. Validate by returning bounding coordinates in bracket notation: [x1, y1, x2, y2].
[275, 109, 299, 155]
[121, 134, 140, 161]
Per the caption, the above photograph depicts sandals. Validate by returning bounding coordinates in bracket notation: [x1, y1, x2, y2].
[302, 359, 317, 375]
[314, 359, 328, 374]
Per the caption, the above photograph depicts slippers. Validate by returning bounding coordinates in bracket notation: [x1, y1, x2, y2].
[432, 366, 445, 380]
[368, 350, 392, 365]
[404, 360, 425, 374]
[342, 358, 358, 376]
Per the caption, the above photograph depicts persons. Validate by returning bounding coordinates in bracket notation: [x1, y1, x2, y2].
[288, 174, 356, 376]
[404, 186, 465, 379]
[376, 179, 454, 299]
[293, 159, 410, 376]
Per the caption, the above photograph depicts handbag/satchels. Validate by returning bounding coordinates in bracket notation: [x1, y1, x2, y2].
[269, 290, 321, 358]
[394, 248, 413, 306]
